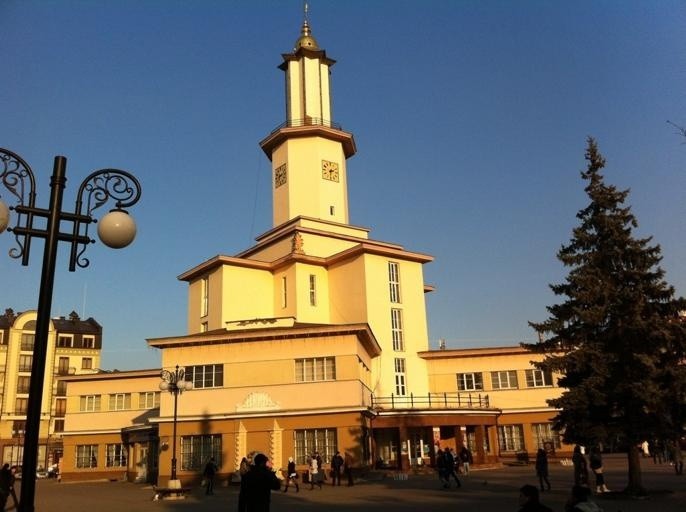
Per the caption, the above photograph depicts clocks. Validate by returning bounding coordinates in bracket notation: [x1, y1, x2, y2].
[274, 163, 286, 188]
[321, 158, 339, 183]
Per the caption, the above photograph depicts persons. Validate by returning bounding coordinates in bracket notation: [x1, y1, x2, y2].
[641, 437, 686, 475]
[204, 458, 218, 496]
[565, 483, 603, 512]
[536, 449, 551, 491]
[238, 455, 280, 512]
[518, 484, 553, 511]
[589, 444, 610, 493]
[573, 446, 590, 484]
[436, 447, 470, 490]
[239, 450, 353, 493]
[0, 463, 19, 512]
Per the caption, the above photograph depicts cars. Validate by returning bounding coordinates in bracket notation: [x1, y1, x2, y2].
[9, 464, 59, 479]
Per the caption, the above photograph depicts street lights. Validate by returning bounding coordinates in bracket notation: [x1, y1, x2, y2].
[160, 364, 194, 500]
[0, 148, 140, 512]
